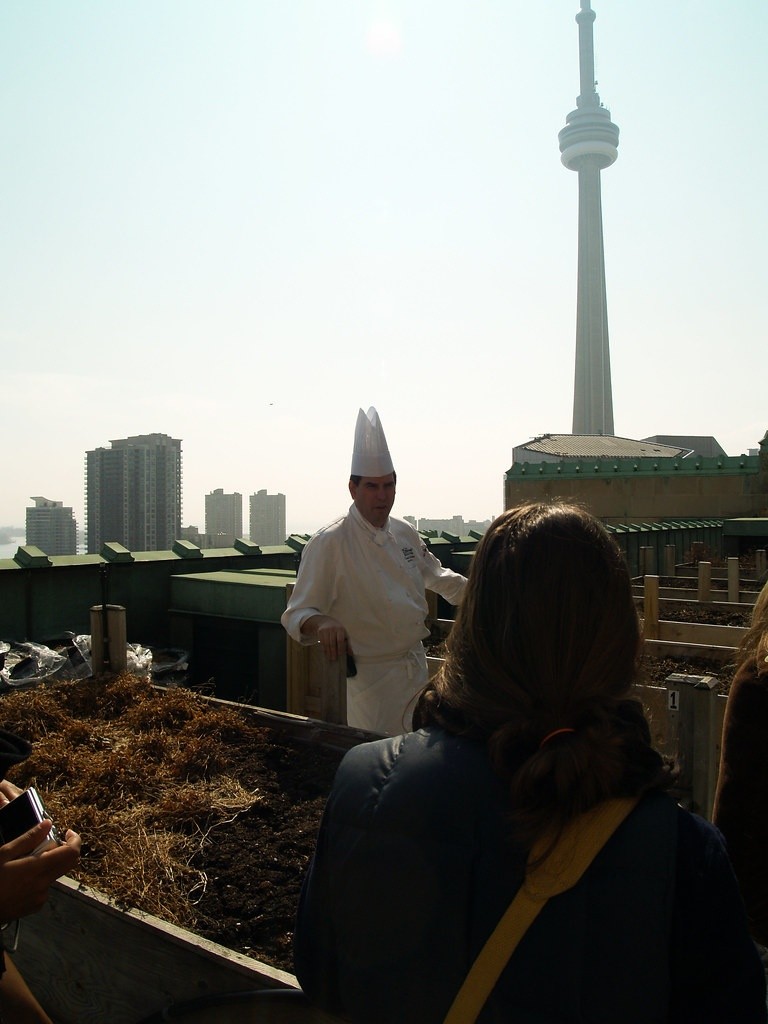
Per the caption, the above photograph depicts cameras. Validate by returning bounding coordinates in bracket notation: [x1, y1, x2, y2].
[0, 785, 62, 863]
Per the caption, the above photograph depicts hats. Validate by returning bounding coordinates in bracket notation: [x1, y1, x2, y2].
[351, 405, 394, 478]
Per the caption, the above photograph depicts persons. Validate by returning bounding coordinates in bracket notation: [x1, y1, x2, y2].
[711, 582, 768, 947]
[293, 503, 768, 1024]
[281, 406, 469, 737]
[0, 778, 82, 1024]
